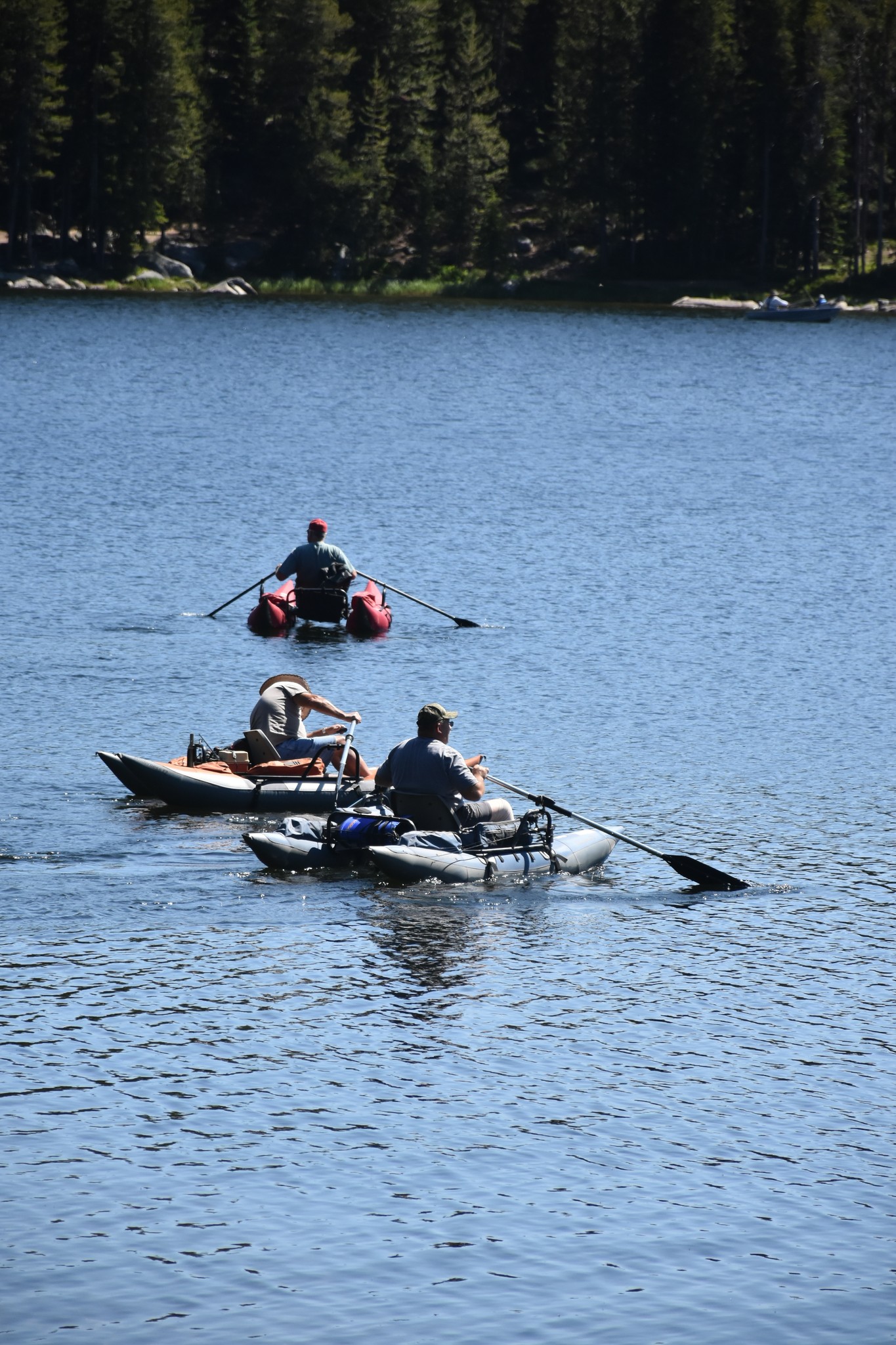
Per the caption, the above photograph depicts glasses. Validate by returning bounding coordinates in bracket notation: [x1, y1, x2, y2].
[439, 721, 454, 727]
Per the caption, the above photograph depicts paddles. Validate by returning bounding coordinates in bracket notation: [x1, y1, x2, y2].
[355, 569, 481, 628]
[466, 765, 752, 892]
[331, 719, 358, 813]
[208, 571, 276, 616]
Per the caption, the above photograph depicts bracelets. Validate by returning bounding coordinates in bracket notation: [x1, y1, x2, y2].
[322, 727, 329, 736]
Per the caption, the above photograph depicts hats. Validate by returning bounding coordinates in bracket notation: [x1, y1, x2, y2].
[416, 704, 459, 724]
[304, 518, 327, 533]
[259, 673, 312, 723]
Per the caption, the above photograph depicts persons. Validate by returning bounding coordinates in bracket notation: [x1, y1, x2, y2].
[374, 703, 515, 831]
[250, 675, 373, 778]
[275, 518, 357, 580]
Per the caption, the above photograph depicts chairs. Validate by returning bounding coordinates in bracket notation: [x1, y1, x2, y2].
[295, 587, 349, 623]
[389, 787, 465, 831]
[241, 728, 283, 764]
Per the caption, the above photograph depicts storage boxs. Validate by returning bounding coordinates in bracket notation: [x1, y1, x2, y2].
[218, 748, 251, 772]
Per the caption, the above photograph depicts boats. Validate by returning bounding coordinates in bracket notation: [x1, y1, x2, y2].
[94, 728, 488, 816]
[246, 578, 392, 634]
[241, 792, 625, 885]
[745, 300, 842, 322]
